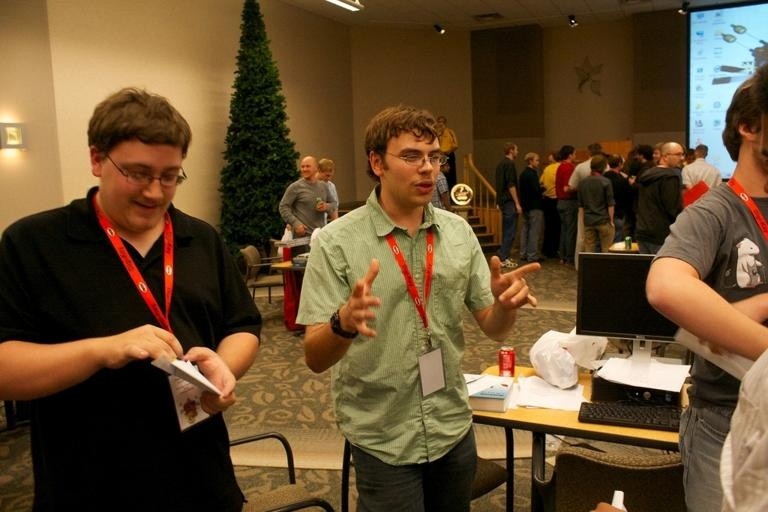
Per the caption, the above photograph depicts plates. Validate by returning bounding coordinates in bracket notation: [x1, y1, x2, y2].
[451, 183, 474, 205]
[292, 260, 306, 267]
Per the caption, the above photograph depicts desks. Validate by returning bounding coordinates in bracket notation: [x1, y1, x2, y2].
[338, 363, 692, 512]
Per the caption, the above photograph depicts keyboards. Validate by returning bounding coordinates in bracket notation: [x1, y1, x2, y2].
[578, 401, 684, 432]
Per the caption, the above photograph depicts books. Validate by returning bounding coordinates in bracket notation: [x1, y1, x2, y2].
[462, 372, 516, 413]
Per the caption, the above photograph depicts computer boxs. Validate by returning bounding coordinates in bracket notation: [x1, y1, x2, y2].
[591, 352, 684, 410]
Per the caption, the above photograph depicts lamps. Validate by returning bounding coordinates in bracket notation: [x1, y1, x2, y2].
[568, 15, 579, 29]
[433, 24, 445, 35]
[678, 0, 690, 15]
[326, 0, 365, 14]
[0, 122, 27, 149]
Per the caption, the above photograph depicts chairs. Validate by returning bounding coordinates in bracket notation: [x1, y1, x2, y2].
[532, 436, 686, 512]
[238, 244, 285, 305]
[223, 429, 334, 511]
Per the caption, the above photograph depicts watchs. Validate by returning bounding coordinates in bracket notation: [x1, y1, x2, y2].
[329, 309, 358, 339]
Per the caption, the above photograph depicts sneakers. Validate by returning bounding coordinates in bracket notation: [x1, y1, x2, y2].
[500, 257, 518, 269]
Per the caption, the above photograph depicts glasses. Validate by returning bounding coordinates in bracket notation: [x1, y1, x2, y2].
[104, 151, 188, 188]
[386, 149, 446, 169]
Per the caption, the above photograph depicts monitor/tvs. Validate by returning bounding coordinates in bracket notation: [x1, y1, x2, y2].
[575, 253, 684, 365]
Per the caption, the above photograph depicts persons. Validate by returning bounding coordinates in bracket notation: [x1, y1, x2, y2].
[433, 115, 459, 190]
[277, 155, 339, 240]
[431, 158, 453, 211]
[286, 157, 340, 233]
[643, 59, 768, 511]
[293, 103, 543, 512]
[495, 140, 722, 272]
[0, 84, 264, 512]
